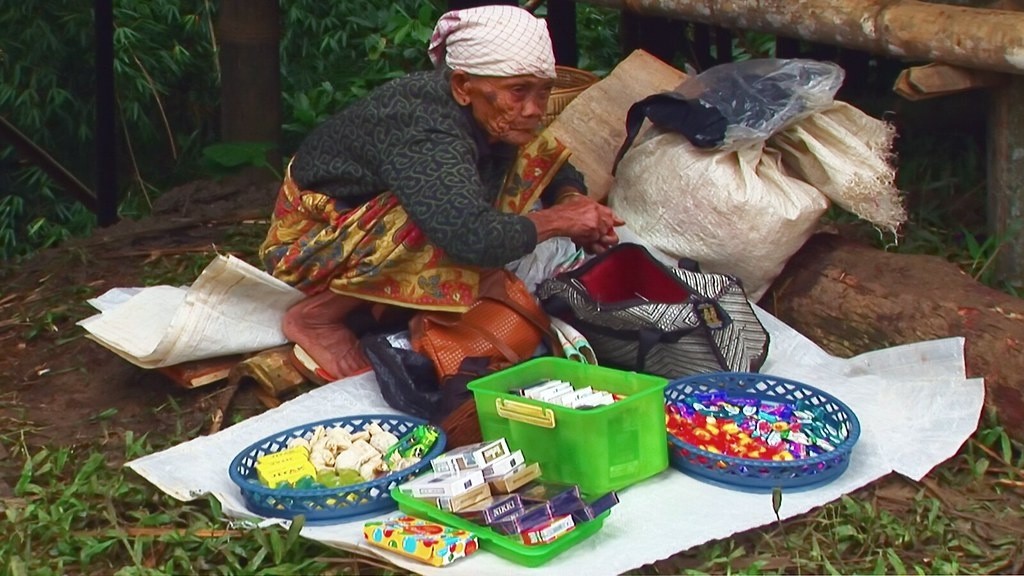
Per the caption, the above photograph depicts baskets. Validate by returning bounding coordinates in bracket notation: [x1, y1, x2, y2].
[658, 370, 862, 496]
[228, 412, 448, 526]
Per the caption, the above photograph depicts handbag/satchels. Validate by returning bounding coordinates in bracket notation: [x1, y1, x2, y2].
[405, 267, 565, 395]
[611, 56, 846, 176]
[536, 241, 771, 384]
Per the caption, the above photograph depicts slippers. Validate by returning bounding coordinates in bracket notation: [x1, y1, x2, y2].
[287, 343, 376, 386]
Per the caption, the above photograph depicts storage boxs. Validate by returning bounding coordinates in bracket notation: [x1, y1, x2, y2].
[466, 355, 668, 494]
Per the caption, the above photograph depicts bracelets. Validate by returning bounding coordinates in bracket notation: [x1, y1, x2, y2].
[553, 191, 583, 205]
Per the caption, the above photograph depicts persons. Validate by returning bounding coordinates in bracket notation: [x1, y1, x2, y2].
[258, 5, 627, 386]
